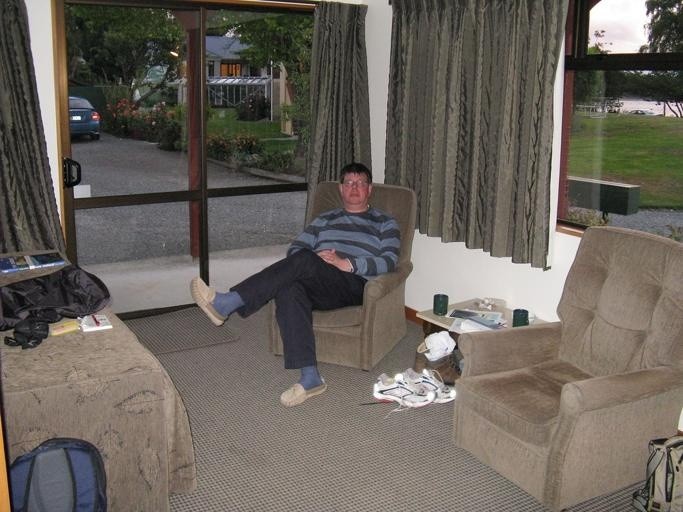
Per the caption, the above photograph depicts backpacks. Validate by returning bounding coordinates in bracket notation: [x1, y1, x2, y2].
[7, 435, 109, 512]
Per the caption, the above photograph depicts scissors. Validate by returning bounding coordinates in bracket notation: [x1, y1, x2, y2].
[362, 399, 392, 405]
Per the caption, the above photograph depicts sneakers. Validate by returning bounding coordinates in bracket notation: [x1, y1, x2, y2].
[278, 373, 328, 410]
[189, 275, 230, 327]
[371, 372, 437, 409]
[402, 366, 458, 406]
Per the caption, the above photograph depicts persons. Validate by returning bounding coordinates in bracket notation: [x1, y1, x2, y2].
[186, 163, 401, 409]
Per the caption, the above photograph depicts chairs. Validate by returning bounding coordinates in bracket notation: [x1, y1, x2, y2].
[269, 178, 419, 374]
[450, 225, 682, 512]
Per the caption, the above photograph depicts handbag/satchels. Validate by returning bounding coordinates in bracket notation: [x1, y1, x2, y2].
[630, 432, 683, 512]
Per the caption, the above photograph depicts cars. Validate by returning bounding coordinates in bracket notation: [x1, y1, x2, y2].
[68, 95, 101, 143]
[622, 108, 663, 118]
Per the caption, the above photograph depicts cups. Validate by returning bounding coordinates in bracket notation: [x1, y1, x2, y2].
[433, 293, 448, 316]
[512, 309, 528, 327]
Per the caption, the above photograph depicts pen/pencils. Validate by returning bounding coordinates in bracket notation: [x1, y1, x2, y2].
[92, 315, 100, 326]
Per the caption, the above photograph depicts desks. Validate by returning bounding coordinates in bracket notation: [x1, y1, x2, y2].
[416, 296, 553, 385]
[1, 308, 198, 511]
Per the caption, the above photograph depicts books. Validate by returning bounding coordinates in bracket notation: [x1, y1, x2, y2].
[24, 252, 65, 270]
[81, 315, 113, 333]
[445, 308, 508, 334]
[0, 256, 29, 274]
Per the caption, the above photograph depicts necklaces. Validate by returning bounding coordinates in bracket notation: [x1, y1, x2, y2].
[345, 204, 369, 213]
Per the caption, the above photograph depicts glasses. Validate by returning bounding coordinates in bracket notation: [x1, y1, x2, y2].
[342, 178, 370, 189]
